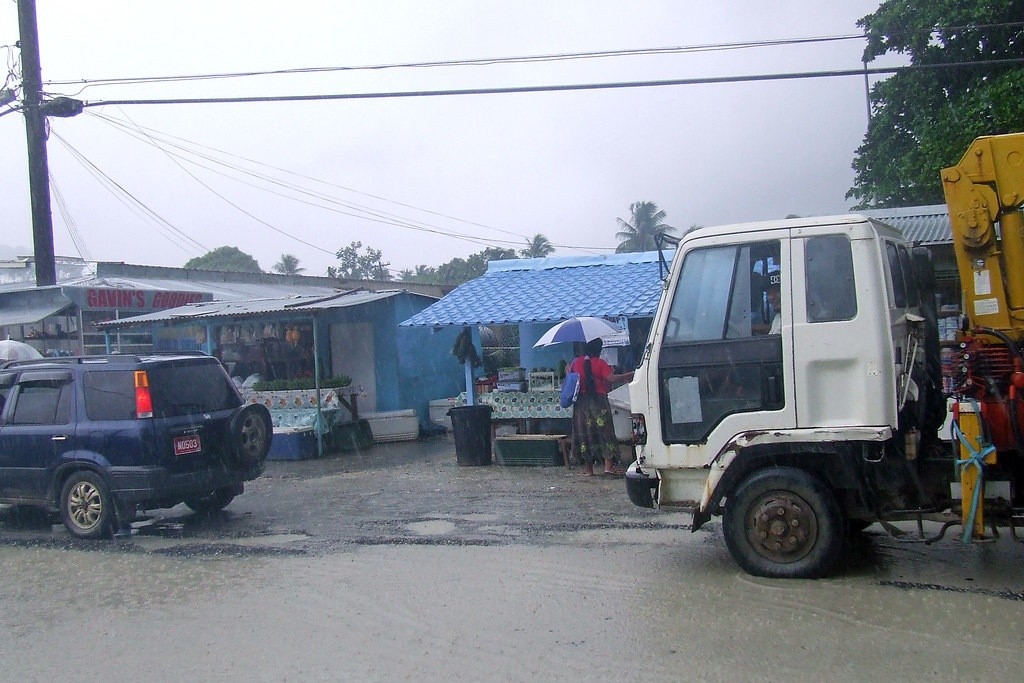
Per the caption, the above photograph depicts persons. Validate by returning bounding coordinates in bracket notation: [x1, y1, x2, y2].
[751, 270, 782, 335]
[567, 337, 634, 475]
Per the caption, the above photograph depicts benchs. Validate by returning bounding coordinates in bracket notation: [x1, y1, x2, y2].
[359, 409, 420, 443]
[429, 396, 454, 432]
[494, 433, 570, 467]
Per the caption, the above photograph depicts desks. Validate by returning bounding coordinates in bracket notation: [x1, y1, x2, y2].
[238, 384, 369, 421]
[455, 391, 576, 459]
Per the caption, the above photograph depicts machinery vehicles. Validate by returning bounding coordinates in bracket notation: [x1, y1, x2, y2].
[605, 129, 1023, 576]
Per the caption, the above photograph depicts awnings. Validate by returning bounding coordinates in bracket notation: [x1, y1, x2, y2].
[394, 259, 673, 404]
[92, 291, 402, 457]
[0, 302, 76, 325]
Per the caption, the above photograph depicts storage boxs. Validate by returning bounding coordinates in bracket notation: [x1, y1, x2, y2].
[266, 425, 317, 460]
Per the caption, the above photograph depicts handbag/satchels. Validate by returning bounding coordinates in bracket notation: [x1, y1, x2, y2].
[559, 357, 583, 408]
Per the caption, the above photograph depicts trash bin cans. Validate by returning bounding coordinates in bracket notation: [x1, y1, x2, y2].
[446, 404, 494, 467]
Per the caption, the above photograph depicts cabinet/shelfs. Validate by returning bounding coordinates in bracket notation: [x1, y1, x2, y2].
[76, 307, 153, 354]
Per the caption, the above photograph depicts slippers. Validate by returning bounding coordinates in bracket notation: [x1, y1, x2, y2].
[581, 472, 596, 477]
[604, 468, 625, 475]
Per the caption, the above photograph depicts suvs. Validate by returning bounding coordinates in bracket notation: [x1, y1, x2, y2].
[2, 349, 273, 535]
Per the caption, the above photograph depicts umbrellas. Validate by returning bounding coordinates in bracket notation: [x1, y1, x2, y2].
[532, 311, 621, 353]
[0, 335, 45, 360]
